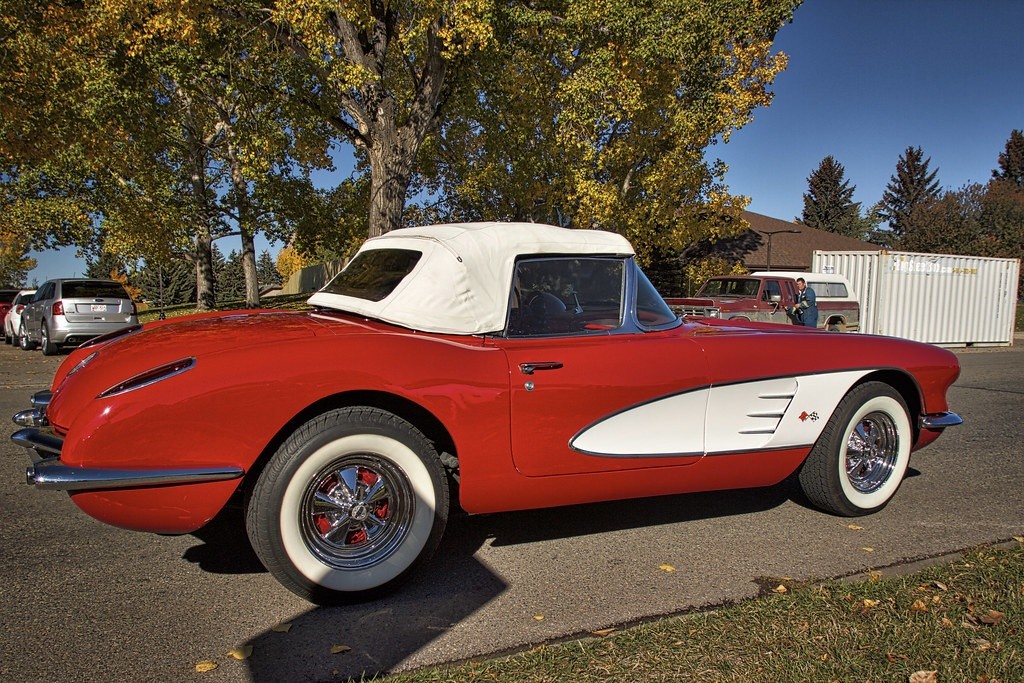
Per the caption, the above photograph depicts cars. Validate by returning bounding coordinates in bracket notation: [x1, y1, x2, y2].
[18, 278, 139, 357]
[2, 289, 38, 347]
[0, 289, 22, 335]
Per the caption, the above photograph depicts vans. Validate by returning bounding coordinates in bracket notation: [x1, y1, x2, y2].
[749, 270, 857, 302]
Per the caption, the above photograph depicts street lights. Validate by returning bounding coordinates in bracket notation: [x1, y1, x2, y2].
[758, 228, 803, 271]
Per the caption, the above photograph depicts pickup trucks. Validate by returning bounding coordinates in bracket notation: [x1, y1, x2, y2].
[658, 275, 860, 334]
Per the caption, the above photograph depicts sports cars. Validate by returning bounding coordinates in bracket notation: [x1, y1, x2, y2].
[8, 222, 966, 611]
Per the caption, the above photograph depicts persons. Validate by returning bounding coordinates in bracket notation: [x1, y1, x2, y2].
[786, 278, 819, 328]
[524, 263, 582, 321]
